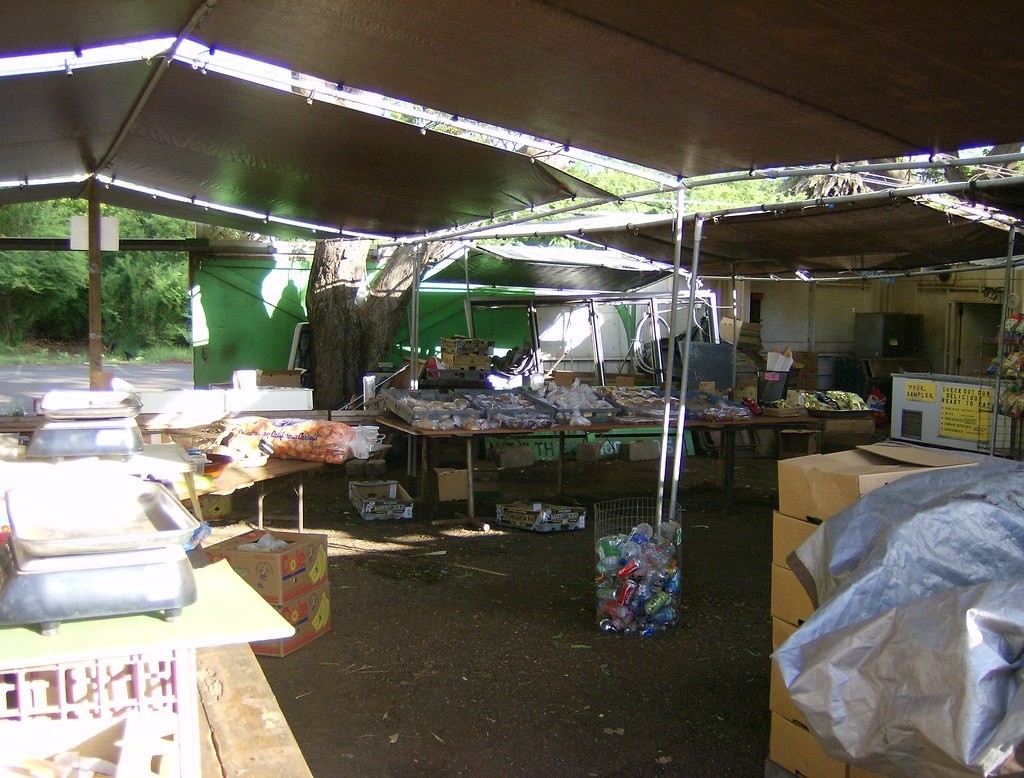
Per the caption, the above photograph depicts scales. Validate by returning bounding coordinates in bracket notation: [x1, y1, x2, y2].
[22, 387, 144, 458]
[0, 476, 206, 629]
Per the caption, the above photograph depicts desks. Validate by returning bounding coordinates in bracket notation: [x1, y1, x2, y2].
[178, 443, 392, 533]
[9, 544, 315, 778]
[375, 415, 821, 526]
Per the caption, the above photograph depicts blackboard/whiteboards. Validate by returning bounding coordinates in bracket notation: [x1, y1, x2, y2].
[678, 340, 735, 390]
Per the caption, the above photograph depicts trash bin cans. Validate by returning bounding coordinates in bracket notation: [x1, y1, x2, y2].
[593, 496, 683, 634]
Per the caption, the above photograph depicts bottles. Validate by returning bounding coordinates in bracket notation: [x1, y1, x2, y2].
[620, 523, 653, 564]
[654, 606, 680, 625]
[665, 569, 680, 605]
[637, 540, 676, 569]
[653, 533, 675, 554]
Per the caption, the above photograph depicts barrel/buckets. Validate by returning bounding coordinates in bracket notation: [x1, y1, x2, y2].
[756, 370, 791, 407]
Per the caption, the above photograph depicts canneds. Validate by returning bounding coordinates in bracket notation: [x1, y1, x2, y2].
[595, 537, 680, 634]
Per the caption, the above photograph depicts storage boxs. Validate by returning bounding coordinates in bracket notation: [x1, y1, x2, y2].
[0, 317, 1024, 778]
